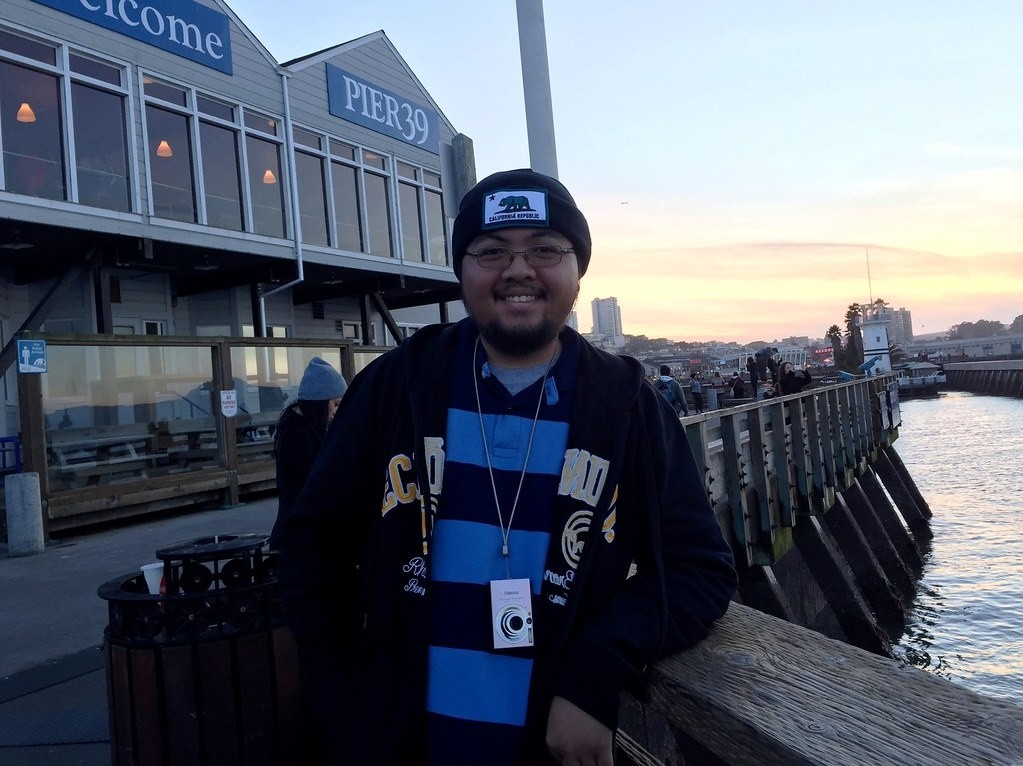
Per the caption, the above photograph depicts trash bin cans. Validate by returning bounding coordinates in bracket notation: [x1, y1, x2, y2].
[97, 532, 312, 766]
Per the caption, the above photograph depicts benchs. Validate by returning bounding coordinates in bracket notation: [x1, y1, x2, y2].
[41, 411, 280, 535]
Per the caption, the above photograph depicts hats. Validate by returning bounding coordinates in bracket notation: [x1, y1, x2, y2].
[298, 357, 348, 401]
[452, 169, 592, 282]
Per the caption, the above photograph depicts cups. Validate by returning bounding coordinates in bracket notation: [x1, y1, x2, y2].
[141, 563, 166, 606]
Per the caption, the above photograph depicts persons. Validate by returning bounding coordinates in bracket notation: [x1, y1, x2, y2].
[269, 356, 349, 560]
[652, 347, 814, 416]
[275, 169, 739, 765]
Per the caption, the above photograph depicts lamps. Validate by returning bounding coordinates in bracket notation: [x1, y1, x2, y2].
[412, 287, 430, 293]
[0, 229, 34, 249]
[194, 254, 219, 270]
[321, 271, 342, 284]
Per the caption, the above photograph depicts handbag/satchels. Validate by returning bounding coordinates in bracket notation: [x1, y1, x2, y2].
[730, 388, 734, 397]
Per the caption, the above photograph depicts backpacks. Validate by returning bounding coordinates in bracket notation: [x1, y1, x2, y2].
[658, 380, 675, 404]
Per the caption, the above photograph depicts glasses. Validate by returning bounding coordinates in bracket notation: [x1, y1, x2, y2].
[465, 244, 578, 269]
